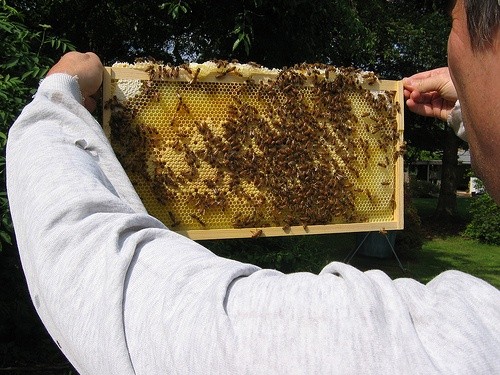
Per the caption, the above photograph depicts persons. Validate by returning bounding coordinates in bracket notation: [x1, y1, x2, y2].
[6, 0, 500, 375]
